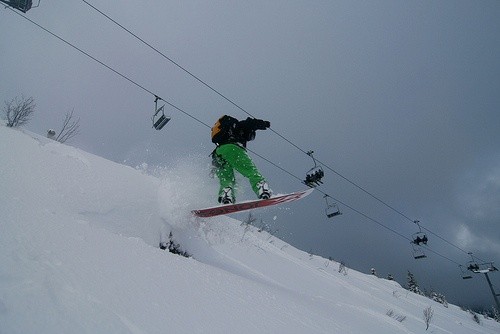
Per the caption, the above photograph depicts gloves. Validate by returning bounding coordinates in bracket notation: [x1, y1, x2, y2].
[263, 120, 270, 128]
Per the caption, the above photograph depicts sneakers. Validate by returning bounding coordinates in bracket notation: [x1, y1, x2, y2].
[222, 187, 234, 204]
[259, 189, 270, 199]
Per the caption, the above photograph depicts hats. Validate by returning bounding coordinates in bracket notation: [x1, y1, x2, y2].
[247, 129, 256, 141]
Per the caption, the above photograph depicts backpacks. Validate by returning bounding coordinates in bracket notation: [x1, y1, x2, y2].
[211, 114, 240, 148]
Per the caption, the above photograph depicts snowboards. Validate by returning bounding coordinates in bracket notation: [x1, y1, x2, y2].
[189, 189, 315, 218]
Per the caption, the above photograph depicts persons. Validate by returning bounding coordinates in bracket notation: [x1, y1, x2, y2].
[415, 236, 420, 245]
[304, 169, 324, 188]
[208, 117, 271, 204]
[422, 235, 428, 244]
[470, 264, 479, 271]
